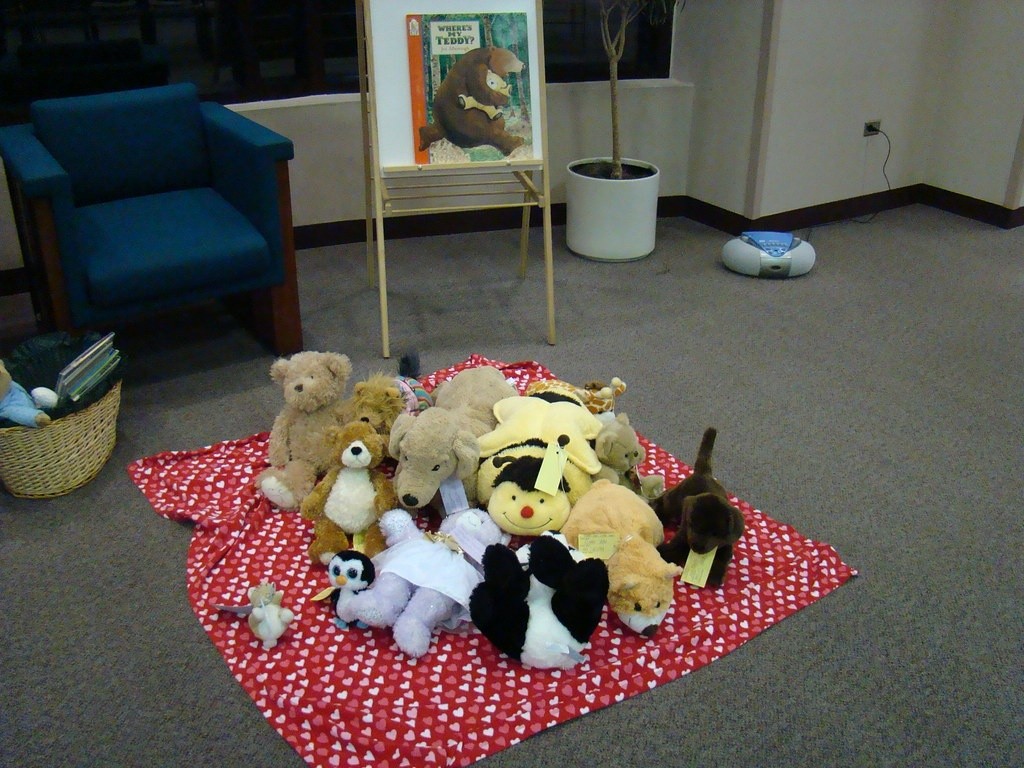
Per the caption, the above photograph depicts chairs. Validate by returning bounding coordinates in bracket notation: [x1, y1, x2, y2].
[0, 84, 303, 357]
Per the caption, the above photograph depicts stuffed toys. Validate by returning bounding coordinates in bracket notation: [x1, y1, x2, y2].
[246, 582, 295, 648]
[255, 351, 744, 670]
[0, 359, 58, 429]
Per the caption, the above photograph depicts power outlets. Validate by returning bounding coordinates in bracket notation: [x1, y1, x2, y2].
[864, 119, 881, 136]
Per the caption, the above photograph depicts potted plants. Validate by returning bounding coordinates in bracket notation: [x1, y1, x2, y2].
[566, 0, 687, 261]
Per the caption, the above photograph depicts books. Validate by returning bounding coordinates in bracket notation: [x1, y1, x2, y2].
[56, 331, 122, 402]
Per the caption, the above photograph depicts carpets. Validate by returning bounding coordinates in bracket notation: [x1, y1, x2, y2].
[124, 355, 859, 768]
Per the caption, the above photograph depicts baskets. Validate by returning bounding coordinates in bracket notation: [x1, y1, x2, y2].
[0, 341, 122, 502]
